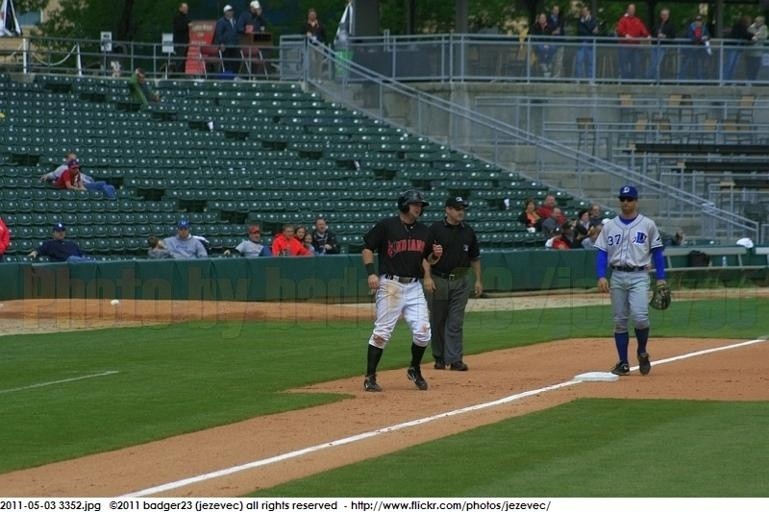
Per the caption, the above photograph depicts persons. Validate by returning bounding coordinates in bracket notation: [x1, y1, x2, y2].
[517, 194, 609, 250]
[147, 236, 171, 259]
[428, 195, 482, 370]
[131, 69, 159, 111]
[593, 185, 667, 375]
[26, 222, 100, 263]
[663, 231, 684, 246]
[237, 1, 269, 45]
[0, 217, 11, 260]
[162, 220, 208, 259]
[362, 188, 443, 390]
[214, 4, 242, 74]
[527, 0, 768, 85]
[40, 152, 116, 198]
[222, 217, 340, 257]
[304, 9, 327, 84]
[168, 2, 192, 79]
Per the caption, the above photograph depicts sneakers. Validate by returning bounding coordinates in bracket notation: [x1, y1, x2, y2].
[435, 359, 445, 369]
[612, 362, 630, 376]
[451, 361, 468, 371]
[406, 366, 428, 390]
[637, 353, 651, 374]
[362, 376, 382, 392]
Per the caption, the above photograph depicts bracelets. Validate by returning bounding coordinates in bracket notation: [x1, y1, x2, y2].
[365, 263, 375, 276]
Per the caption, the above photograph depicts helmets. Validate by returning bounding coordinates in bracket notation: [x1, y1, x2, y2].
[399, 189, 429, 216]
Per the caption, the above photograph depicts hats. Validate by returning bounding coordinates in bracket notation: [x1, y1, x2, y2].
[445, 194, 468, 207]
[177, 220, 189, 230]
[250, 0, 261, 9]
[68, 160, 80, 169]
[223, 5, 234, 12]
[618, 185, 637, 199]
[248, 224, 260, 234]
[52, 223, 65, 231]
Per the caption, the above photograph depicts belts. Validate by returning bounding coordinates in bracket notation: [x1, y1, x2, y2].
[385, 274, 418, 284]
[612, 265, 646, 273]
[432, 271, 464, 280]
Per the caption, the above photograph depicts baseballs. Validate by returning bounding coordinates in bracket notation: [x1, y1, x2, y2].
[110, 298, 119, 306]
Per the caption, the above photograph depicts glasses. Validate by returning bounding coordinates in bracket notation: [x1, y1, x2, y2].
[619, 197, 635, 202]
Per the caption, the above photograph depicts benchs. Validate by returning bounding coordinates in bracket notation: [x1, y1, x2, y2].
[649, 245, 769, 290]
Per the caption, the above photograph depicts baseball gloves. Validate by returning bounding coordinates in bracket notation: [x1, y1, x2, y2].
[649, 283, 671, 310]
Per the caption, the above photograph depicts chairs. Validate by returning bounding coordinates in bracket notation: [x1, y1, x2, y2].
[0, 31, 769, 265]
